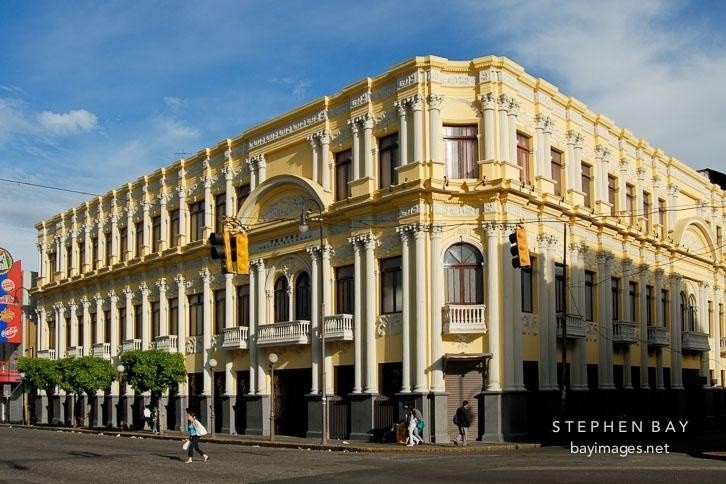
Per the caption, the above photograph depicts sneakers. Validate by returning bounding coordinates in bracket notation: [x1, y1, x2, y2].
[453, 439, 458, 446]
[406, 440, 425, 447]
[184, 455, 209, 464]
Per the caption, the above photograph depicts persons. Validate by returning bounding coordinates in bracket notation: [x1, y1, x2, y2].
[151, 406, 160, 435]
[451, 400, 474, 447]
[183, 411, 210, 463]
[409, 406, 425, 444]
[405, 408, 423, 447]
[142, 404, 152, 432]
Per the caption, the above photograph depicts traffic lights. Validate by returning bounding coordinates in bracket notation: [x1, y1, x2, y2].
[508, 224, 531, 269]
[209, 231, 232, 274]
[230, 234, 249, 274]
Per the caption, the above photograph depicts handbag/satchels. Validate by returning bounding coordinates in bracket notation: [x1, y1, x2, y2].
[453, 414, 457, 424]
[182, 438, 192, 451]
[192, 419, 208, 437]
[417, 419, 424, 431]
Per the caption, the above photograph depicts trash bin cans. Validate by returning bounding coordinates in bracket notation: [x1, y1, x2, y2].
[396, 424, 407, 444]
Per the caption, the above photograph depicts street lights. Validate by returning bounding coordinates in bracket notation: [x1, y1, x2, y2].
[268, 352, 278, 442]
[9, 286, 33, 427]
[208, 357, 218, 438]
[298, 197, 331, 444]
[117, 365, 125, 430]
